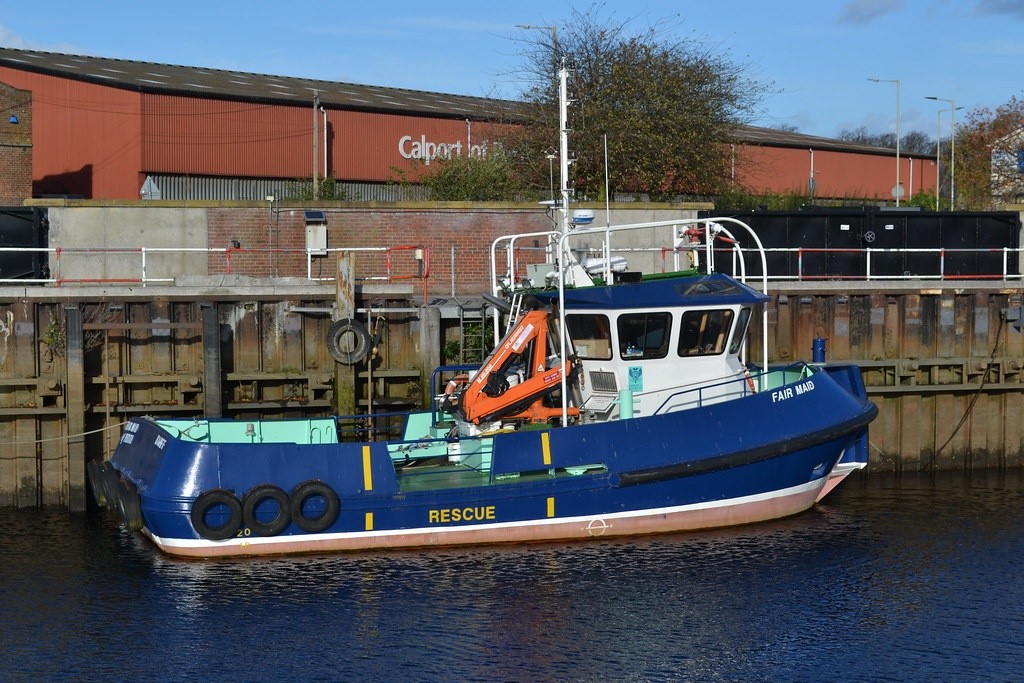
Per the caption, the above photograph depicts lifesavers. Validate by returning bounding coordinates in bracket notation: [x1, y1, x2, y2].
[119, 491, 143, 531]
[445, 373, 470, 393]
[100, 470, 120, 511]
[244, 485, 291, 536]
[745, 369, 756, 394]
[87, 458, 106, 506]
[192, 489, 244, 541]
[326, 318, 370, 364]
[291, 482, 340, 531]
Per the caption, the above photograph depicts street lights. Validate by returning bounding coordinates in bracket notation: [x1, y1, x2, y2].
[936, 105, 966, 213]
[866, 77, 900, 207]
[926, 97, 956, 213]
[516, 24, 555, 94]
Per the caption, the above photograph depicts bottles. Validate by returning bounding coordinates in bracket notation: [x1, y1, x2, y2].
[627, 347, 632, 354]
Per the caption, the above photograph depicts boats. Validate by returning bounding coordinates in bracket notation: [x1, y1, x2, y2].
[544, 256, 628, 288]
[100, 62, 879, 559]
